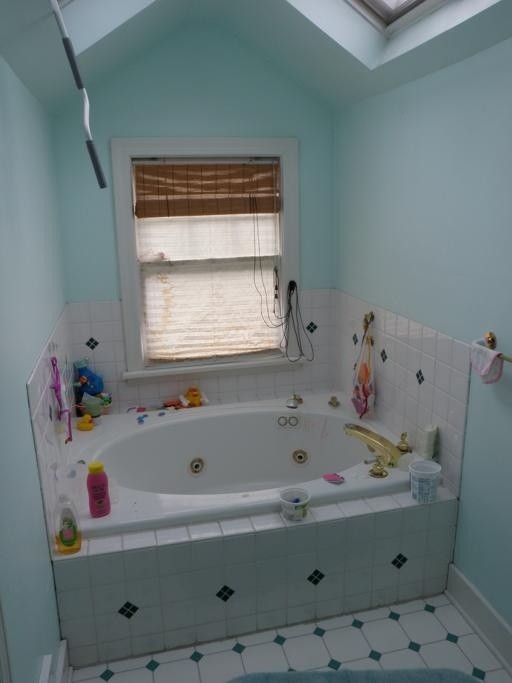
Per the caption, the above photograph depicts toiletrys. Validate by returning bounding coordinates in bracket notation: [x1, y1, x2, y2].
[86, 462, 111, 517]
[53, 494, 81, 554]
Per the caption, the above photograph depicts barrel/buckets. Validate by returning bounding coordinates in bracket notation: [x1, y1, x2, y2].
[409, 460, 441, 503]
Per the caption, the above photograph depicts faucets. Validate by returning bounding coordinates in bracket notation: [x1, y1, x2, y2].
[342, 423, 402, 467]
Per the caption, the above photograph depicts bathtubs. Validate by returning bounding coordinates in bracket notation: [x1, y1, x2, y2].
[57, 392, 424, 538]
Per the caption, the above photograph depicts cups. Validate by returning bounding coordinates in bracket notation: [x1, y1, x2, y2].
[408, 459, 443, 506]
